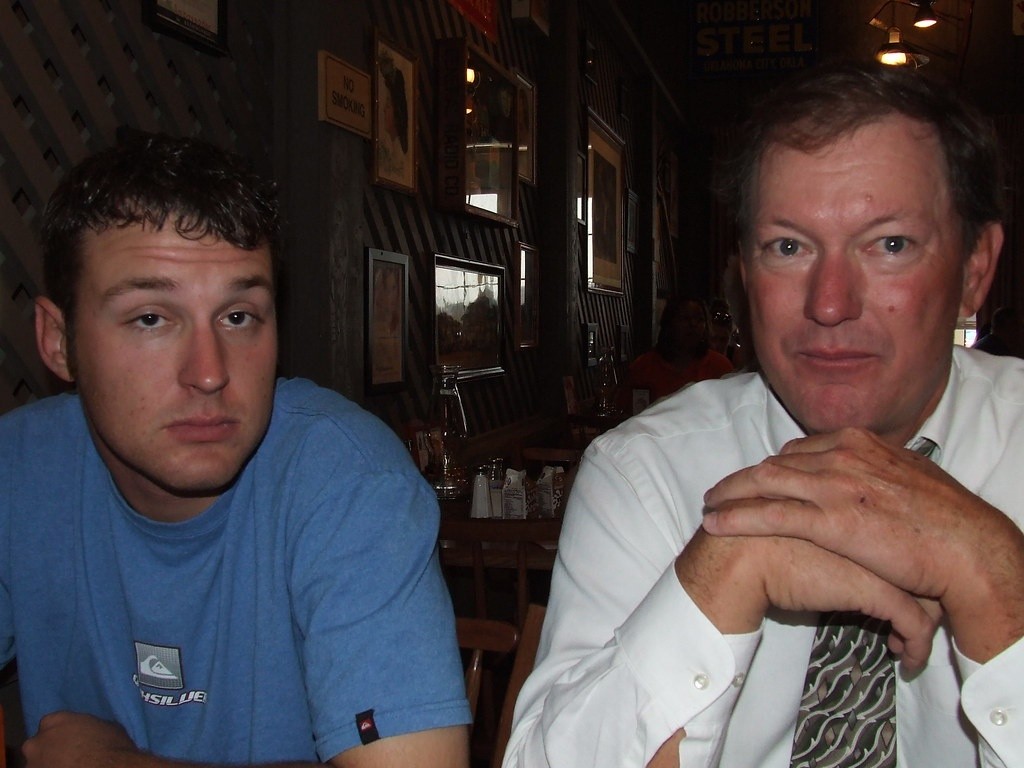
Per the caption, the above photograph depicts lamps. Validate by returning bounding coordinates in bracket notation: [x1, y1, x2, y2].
[913, 0, 938, 28]
[873, 0, 929, 71]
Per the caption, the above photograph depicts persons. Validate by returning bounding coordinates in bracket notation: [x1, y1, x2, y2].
[627, 300, 734, 396]
[0, 133, 475, 768]
[501, 70, 1024, 768]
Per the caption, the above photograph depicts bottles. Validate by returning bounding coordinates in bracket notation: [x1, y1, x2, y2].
[470, 466, 491, 518]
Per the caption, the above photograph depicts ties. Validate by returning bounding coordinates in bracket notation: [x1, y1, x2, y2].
[789, 438, 937, 768]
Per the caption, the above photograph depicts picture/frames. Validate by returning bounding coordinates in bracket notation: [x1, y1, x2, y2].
[142, 0, 230, 58]
[576, 149, 587, 226]
[429, 252, 510, 383]
[372, 27, 422, 198]
[619, 84, 631, 122]
[586, 40, 599, 87]
[584, 106, 627, 298]
[513, 240, 539, 351]
[516, 69, 539, 189]
[364, 247, 409, 393]
[625, 189, 640, 255]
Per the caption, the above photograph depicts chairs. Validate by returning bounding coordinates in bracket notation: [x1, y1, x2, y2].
[439, 515, 561, 759]
[506, 438, 582, 473]
